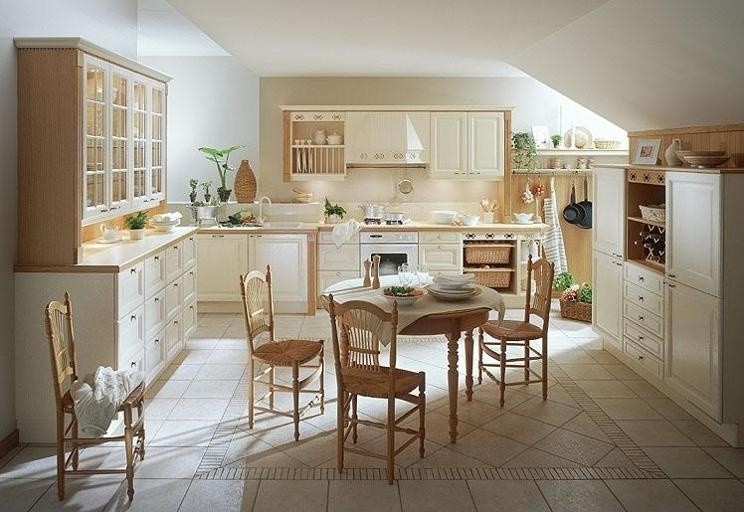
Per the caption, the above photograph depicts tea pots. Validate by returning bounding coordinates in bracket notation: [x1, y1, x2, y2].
[513, 212, 534, 222]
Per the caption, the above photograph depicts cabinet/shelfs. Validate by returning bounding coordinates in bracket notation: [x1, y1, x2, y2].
[194, 230, 318, 317]
[13, 37, 167, 269]
[432, 110, 503, 178]
[15, 226, 196, 447]
[319, 230, 359, 311]
[517, 231, 542, 295]
[420, 230, 460, 279]
[282, 110, 346, 180]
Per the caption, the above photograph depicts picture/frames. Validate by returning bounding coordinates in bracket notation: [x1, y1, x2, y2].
[633, 139, 661, 165]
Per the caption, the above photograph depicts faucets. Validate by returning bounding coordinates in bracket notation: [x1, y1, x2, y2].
[259, 196, 272, 223]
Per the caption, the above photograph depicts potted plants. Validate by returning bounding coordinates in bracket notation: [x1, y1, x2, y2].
[202, 181, 211, 202]
[199, 144, 246, 203]
[189, 179, 197, 203]
[551, 135, 561, 148]
[512, 130, 537, 169]
[124, 211, 149, 241]
[323, 198, 344, 223]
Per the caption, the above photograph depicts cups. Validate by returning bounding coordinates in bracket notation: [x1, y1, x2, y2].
[398, 263, 429, 285]
[360, 232, 418, 278]
[484, 213, 494, 224]
[104, 230, 116, 240]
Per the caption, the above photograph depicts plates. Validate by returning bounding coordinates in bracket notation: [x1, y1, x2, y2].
[565, 126, 593, 151]
[425, 283, 481, 301]
[97, 239, 118, 244]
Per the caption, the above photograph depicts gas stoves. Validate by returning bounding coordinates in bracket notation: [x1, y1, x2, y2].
[360, 218, 403, 225]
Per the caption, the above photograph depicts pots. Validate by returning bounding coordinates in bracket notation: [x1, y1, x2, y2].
[387, 213, 405, 221]
[357, 202, 384, 220]
[327, 133, 341, 145]
[563, 175, 592, 230]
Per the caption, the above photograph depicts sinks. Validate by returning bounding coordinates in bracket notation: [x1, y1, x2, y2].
[261, 222, 303, 228]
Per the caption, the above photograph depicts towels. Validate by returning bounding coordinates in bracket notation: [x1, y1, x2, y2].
[331, 218, 363, 250]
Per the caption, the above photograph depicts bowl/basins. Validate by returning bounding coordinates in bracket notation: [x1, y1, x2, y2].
[296, 198, 312, 203]
[382, 286, 428, 305]
[676, 150, 727, 164]
[460, 214, 481, 226]
[684, 156, 730, 168]
[431, 211, 455, 224]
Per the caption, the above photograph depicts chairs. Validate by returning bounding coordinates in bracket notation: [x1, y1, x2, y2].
[323, 293, 427, 485]
[238, 266, 323, 442]
[38, 289, 146, 505]
[481, 259, 558, 408]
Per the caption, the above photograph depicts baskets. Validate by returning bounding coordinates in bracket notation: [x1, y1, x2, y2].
[594, 140, 619, 149]
[464, 243, 514, 264]
[465, 268, 514, 287]
[639, 204, 665, 223]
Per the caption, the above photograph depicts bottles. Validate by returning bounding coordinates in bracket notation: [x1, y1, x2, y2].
[363, 259, 372, 287]
[373, 255, 381, 289]
